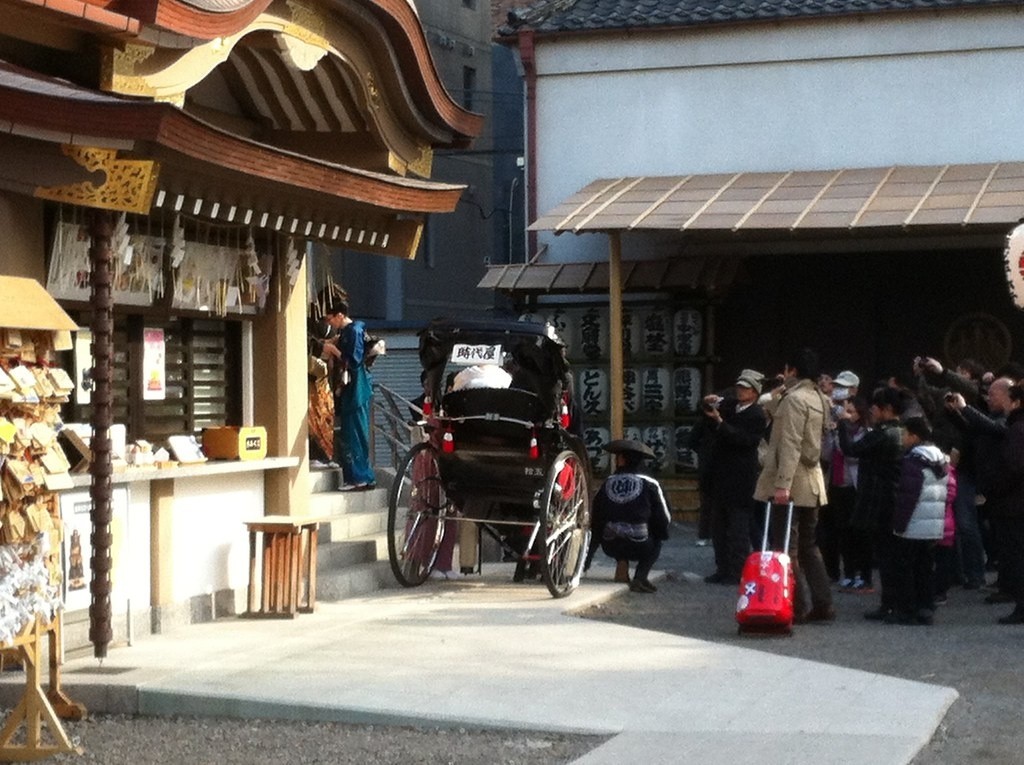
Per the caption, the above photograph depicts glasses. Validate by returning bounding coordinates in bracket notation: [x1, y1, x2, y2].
[326, 314, 337, 324]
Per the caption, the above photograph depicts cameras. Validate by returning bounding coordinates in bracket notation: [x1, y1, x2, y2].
[918, 359, 929, 368]
[945, 395, 955, 402]
[703, 401, 719, 412]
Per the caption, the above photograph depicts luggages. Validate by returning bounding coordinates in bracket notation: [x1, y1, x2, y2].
[735, 494, 796, 635]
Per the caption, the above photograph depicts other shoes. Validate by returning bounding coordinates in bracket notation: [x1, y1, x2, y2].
[310, 459, 339, 468]
[792, 603, 836, 625]
[863, 590, 948, 626]
[696, 539, 713, 546]
[963, 558, 1024, 624]
[429, 569, 458, 581]
[838, 573, 869, 589]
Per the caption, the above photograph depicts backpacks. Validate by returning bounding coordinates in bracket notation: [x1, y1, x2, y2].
[362, 328, 386, 369]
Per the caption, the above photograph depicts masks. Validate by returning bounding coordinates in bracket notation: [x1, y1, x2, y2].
[833, 386, 850, 400]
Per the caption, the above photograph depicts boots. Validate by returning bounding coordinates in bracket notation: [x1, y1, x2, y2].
[614, 559, 630, 583]
[630, 565, 657, 593]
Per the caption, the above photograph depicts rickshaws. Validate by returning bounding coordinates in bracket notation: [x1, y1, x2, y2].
[388, 315, 596, 598]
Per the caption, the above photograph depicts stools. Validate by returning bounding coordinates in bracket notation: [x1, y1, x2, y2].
[238, 515, 325, 619]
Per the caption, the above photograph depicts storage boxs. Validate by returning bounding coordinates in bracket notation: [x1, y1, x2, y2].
[201, 425, 267, 461]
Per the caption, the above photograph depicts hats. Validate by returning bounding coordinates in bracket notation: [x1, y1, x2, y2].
[601, 440, 657, 459]
[832, 370, 860, 387]
[736, 369, 765, 394]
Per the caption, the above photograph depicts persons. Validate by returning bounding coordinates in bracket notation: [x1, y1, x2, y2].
[319, 299, 376, 490]
[690, 352, 1024, 626]
[400, 366, 480, 581]
[580, 440, 671, 594]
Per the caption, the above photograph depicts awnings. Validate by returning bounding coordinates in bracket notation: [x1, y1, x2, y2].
[524, 158, 1024, 237]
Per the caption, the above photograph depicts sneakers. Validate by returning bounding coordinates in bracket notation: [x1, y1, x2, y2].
[338, 481, 376, 491]
[704, 570, 740, 584]
